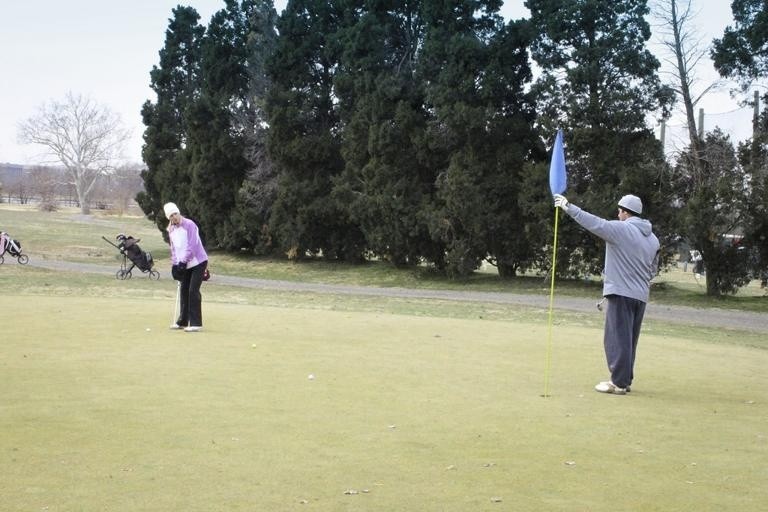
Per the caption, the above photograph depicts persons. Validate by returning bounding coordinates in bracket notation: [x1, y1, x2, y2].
[550, 191, 662, 396]
[161, 199, 209, 332]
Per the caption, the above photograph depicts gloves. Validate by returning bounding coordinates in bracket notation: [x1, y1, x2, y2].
[172, 265, 182, 281]
[176, 261, 186, 274]
[553, 193, 570, 211]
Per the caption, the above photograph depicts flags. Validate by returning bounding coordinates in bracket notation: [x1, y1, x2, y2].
[545, 128, 572, 199]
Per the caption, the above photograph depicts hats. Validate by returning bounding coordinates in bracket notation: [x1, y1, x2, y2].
[164, 202, 181, 220]
[617, 195, 643, 215]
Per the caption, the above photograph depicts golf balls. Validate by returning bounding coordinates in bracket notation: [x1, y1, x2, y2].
[146, 327, 151, 332]
[252, 344, 257, 348]
[307, 374, 315, 380]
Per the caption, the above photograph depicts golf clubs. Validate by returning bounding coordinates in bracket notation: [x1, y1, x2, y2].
[116, 233, 127, 241]
[597, 235, 682, 311]
[173, 281, 181, 328]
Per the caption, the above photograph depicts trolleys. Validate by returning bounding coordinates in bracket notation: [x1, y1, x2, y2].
[1, 232, 29, 265]
[104, 235, 161, 281]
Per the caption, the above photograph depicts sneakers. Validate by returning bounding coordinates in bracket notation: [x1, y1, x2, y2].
[170, 324, 188, 329]
[593, 383, 626, 395]
[184, 326, 201, 332]
[597, 381, 632, 391]
